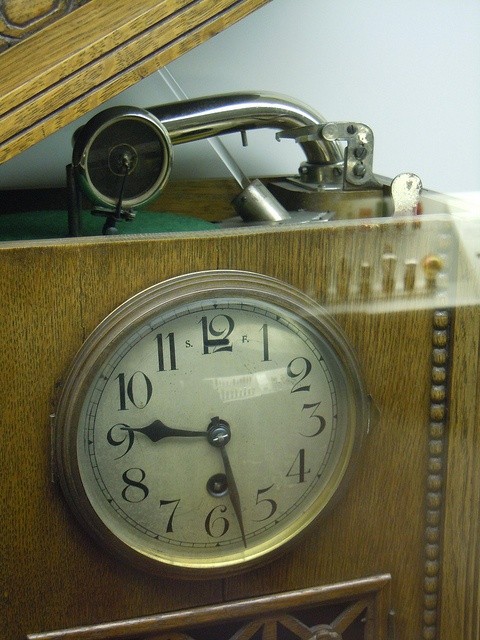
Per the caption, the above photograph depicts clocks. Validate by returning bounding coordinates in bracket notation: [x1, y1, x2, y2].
[1, 0, 478, 640]
